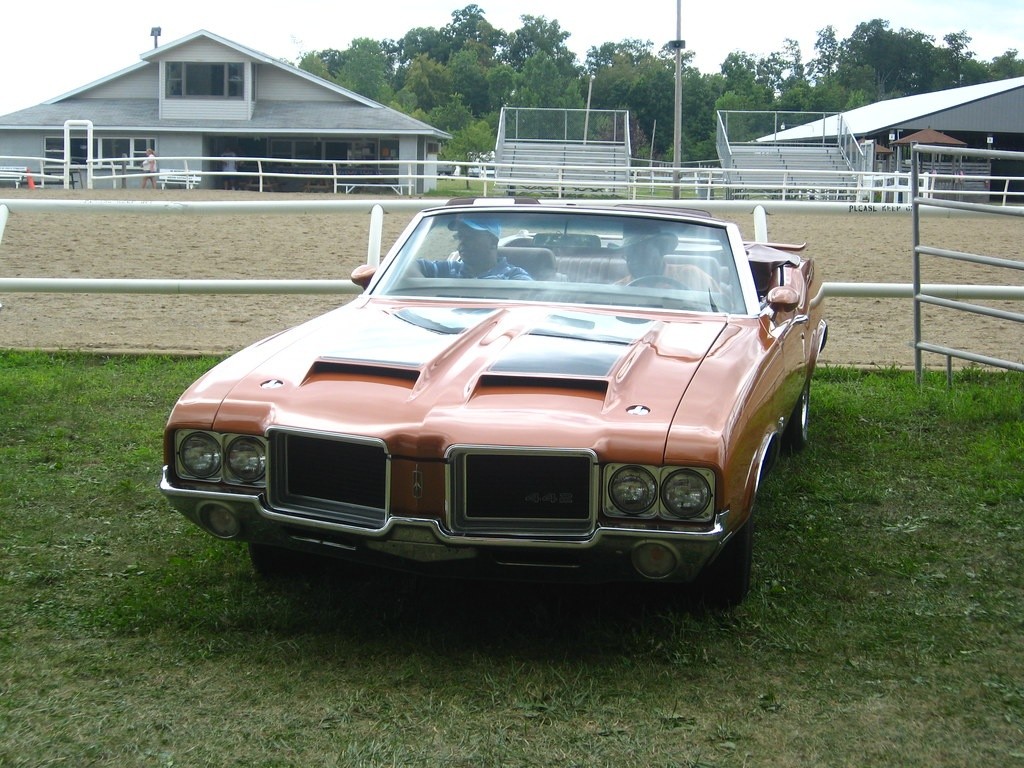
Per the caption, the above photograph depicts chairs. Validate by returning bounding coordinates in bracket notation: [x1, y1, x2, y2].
[497, 247, 558, 282]
[663, 255, 722, 292]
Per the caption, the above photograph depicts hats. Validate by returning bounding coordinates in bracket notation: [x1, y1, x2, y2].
[607, 223, 680, 256]
[447, 217, 501, 240]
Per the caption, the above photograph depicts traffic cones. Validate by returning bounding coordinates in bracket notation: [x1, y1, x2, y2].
[23, 169, 36, 192]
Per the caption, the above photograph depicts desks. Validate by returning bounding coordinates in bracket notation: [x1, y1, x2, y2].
[27, 169, 83, 190]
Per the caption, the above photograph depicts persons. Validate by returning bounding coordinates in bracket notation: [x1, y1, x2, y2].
[220, 147, 244, 190]
[407, 216, 536, 281]
[141, 148, 157, 189]
[606, 221, 720, 292]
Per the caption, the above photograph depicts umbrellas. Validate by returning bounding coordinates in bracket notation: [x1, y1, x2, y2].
[889, 124, 967, 173]
[843, 137, 894, 153]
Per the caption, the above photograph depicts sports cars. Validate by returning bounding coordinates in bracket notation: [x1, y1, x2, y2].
[156, 197, 832, 608]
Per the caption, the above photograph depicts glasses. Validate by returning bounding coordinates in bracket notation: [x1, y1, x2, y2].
[622, 249, 655, 261]
[453, 231, 491, 240]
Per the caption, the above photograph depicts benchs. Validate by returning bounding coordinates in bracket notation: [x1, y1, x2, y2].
[336, 184, 414, 196]
[725, 141, 859, 200]
[156, 168, 202, 190]
[16, 180, 79, 184]
[493, 138, 631, 199]
[0, 166, 28, 189]
[552, 253, 734, 301]
